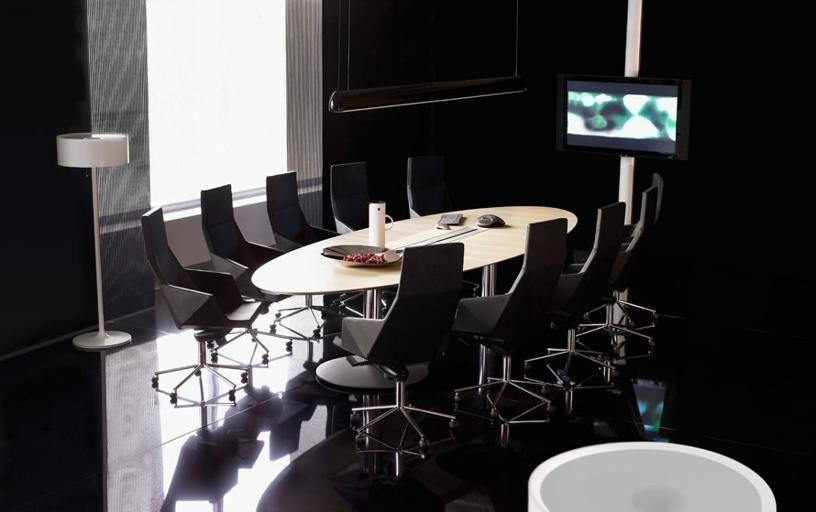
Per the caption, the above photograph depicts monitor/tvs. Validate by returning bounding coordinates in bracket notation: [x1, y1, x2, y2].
[589, 375, 683, 442]
[562, 75, 683, 160]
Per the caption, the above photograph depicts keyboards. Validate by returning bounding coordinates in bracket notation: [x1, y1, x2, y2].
[438, 214, 463, 224]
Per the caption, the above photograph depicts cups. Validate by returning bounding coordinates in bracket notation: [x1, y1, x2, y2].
[368, 201, 394, 247]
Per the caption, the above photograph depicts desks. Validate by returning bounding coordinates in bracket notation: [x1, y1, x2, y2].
[527, 441, 777, 512]
[252, 204, 579, 392]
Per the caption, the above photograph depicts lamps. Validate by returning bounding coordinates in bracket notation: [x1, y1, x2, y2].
[56, 131, 133, 349]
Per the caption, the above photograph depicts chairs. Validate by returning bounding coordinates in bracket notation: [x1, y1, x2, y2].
[330, 163, 397, 319]
[406, 143, 481, 300]
[142, 206, 269, 408]
[452, 217, 571, 447]
[569, 186, 656, 354]
[547, 201, 628, 385]
[583, 169, 663, 332]
[332, 243, 459, 477]
[265, 171, 348, 342]
[199, 184, 321, 353]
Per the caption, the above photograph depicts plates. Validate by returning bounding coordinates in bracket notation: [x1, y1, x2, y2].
[320, 245, 403, 267]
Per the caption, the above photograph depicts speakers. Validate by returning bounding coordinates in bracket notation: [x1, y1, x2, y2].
[555, 73, 565, 151]
[678, 79, 692, 160]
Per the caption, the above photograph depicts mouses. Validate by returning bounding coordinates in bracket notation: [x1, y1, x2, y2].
[436, 223, 449, 230]
